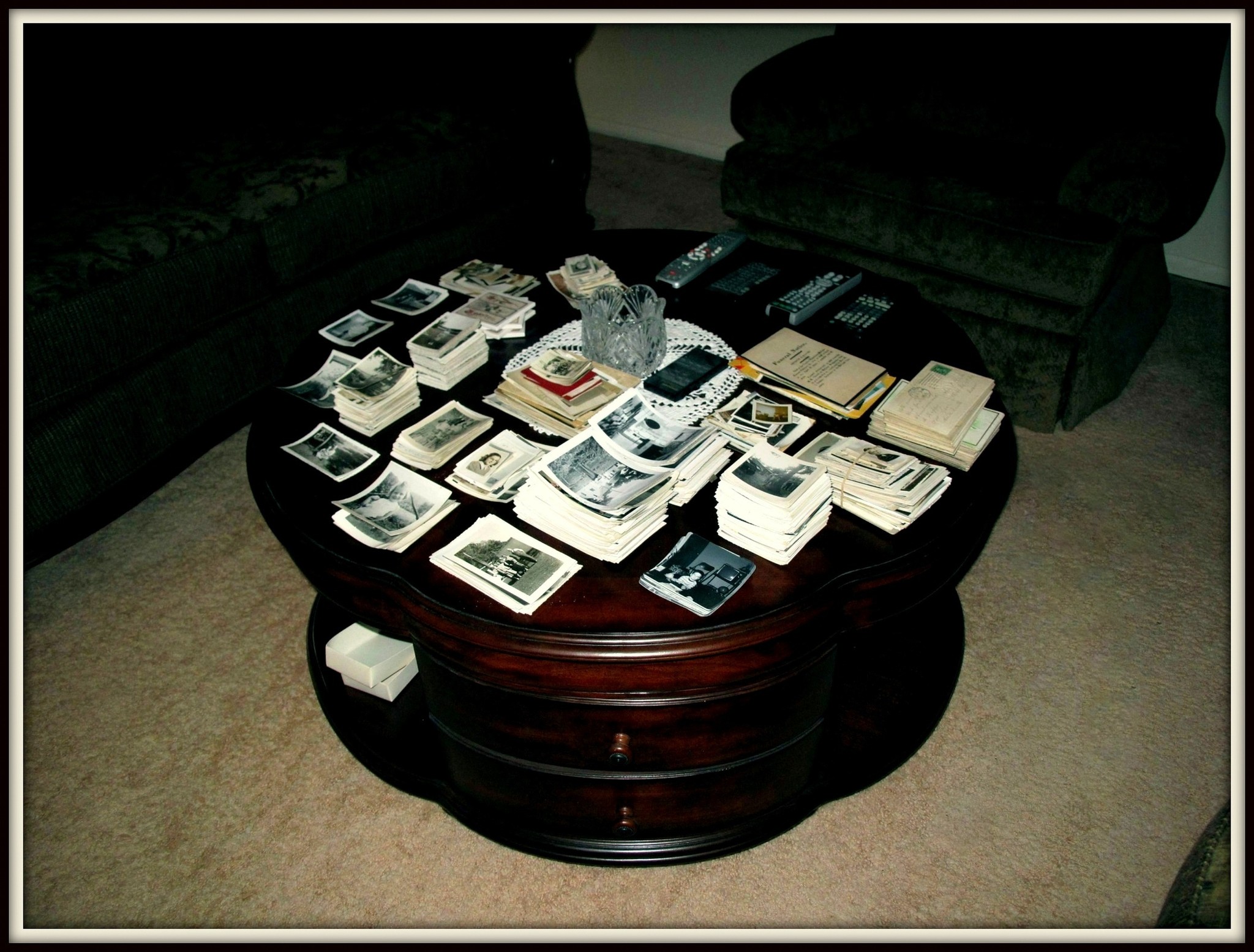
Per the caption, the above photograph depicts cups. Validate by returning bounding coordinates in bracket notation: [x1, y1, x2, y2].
[579, 284, 666, 378]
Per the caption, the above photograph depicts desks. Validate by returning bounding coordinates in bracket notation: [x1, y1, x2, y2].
[245, 226, 1021, 868]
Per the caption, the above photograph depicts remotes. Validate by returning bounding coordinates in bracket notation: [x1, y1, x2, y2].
[654, 231, 906, 335]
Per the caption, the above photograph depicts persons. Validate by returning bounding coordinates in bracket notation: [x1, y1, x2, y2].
[295, 255, 824, 615]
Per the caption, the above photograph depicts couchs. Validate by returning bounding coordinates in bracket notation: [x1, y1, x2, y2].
[724, 25, 1230, 428]
[25, 26, 601, 567]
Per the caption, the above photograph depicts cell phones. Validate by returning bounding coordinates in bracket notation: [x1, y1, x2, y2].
[644, 348, 728, 401]
